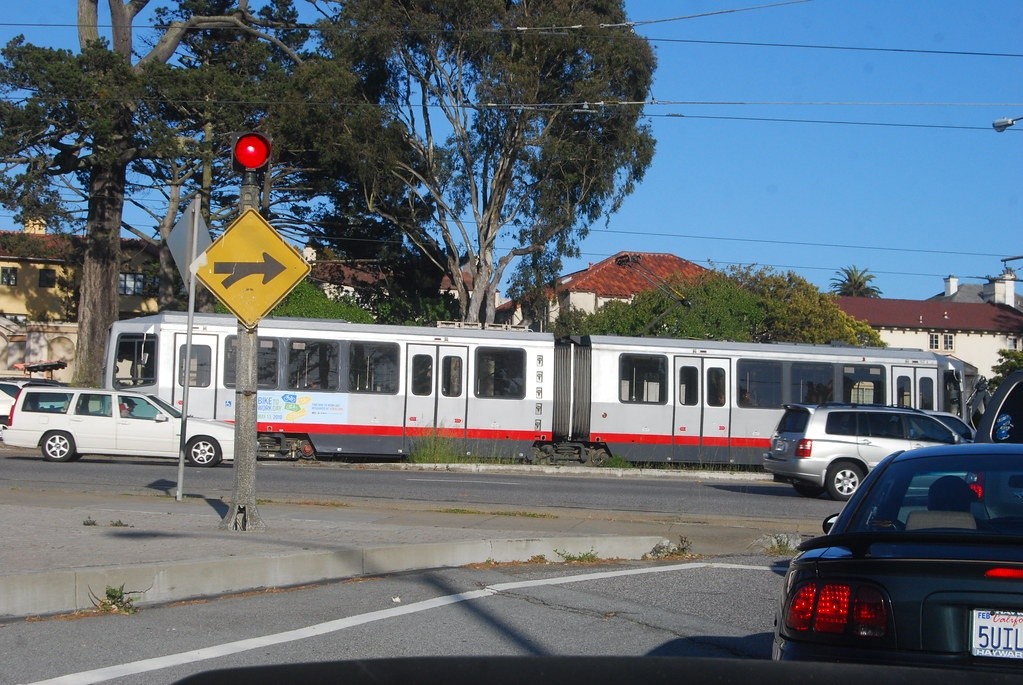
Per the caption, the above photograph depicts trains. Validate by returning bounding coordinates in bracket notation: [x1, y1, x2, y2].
[103, 309, 981, 469]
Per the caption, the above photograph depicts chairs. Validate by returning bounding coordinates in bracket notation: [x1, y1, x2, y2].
[903, 510, 975, 531]
[107, 402, 112, 416]
[886, 420, 899, 438]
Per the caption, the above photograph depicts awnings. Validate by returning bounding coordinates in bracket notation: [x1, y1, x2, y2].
[26, 359, 67, 381]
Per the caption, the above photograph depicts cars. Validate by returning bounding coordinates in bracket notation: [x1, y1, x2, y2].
[3, 386, 260, 467]
[767, 444, 1023, 672]
[923, 409, 976, 443]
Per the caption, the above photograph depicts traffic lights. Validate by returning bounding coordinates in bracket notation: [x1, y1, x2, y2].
[230, 130, 272, 185]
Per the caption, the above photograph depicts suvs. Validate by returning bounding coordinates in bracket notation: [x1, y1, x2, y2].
[763, 402, 969, 502]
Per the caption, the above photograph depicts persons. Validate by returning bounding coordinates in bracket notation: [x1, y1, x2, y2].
[120, 400, 138, 418]
[927, 476, 984, 522]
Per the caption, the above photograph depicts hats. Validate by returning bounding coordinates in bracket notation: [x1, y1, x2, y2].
[120, 402, 129, 409]
[123, 398, 138, 405]
